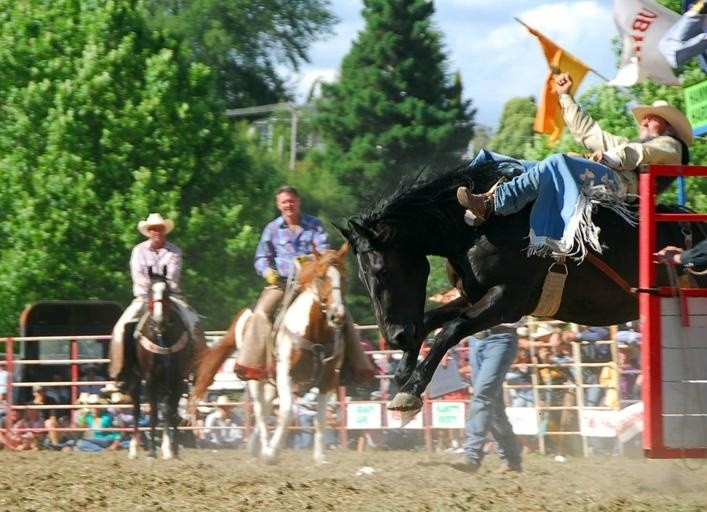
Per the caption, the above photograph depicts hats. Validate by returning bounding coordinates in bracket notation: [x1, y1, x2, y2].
[532, 324, 556, 339]
[213, 395, 235, 405]
[137, 213, 175, 237]
[631, 99, 693, 145]
[32, 386, 43, 391]
[77, 384, 131, 404]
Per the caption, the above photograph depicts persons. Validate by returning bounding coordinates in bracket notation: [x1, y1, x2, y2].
[457, 73, 694, 228]
[1, 383, 357, 453]
[452, 316, 526, 476]
[348, 321, 641, 453]
[232, 185, 354, 383]
[108, 212, 206, 391]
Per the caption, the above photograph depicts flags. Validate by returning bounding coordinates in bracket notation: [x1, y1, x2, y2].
[605, 0, 684, 86]
[526, 28, 590, 145]
[661, 0, 707, 68]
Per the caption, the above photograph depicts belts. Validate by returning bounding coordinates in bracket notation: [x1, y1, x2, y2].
[475, 325, 516, 339]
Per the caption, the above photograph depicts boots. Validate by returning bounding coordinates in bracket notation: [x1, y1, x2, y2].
[457, 176, 504, 227]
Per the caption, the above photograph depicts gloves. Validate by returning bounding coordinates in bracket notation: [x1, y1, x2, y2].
[299, 255, 308, 264]
[265, 270, 280, 284]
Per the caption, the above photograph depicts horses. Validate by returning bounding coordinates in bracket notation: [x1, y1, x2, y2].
[116, 264, 191, 462]
[173, 239, 350, 467]
[330, 153, 706, 427]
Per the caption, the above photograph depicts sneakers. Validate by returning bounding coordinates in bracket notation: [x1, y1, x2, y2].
[353, 380, 380, 390]
[452, 459, 478, 473]
[499, 463, 522, 474]
[234, 362, 266, 379]
